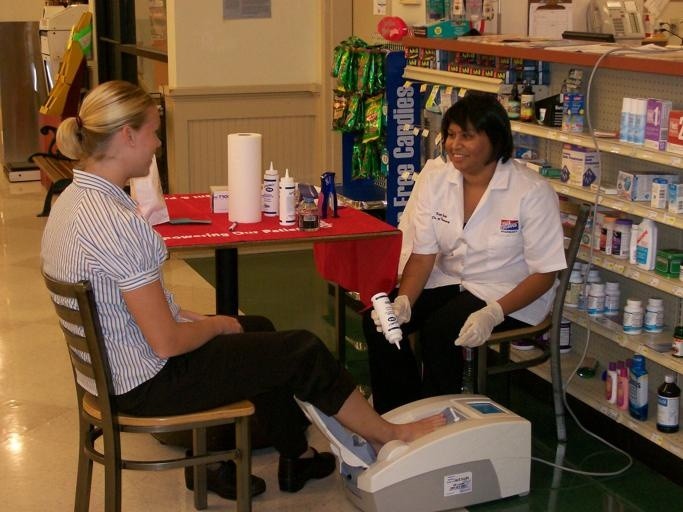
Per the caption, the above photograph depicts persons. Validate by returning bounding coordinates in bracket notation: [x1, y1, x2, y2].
[41, 80, 447, 499]
[369, 90, 567, 418]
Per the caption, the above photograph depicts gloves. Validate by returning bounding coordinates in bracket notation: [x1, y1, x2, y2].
[371, 295, 412, 334]
[455, 301, 505, 348]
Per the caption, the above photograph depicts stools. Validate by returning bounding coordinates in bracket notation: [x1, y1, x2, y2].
[28, 124, 77, 218]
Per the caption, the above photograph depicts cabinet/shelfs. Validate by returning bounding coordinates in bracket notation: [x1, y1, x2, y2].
[402, 32, 682, 455]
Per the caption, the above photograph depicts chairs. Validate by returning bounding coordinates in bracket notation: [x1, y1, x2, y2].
[458, 197, 592, 443]
[38, 262, 256, 512]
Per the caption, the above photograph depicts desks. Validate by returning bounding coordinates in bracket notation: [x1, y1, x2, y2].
[131, 187, 402, 451]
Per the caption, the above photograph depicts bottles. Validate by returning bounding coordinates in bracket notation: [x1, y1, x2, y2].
[371, 292, 404, 350]
[619, 97, 631, 143]
[605, 282, 620, 316]
[580, 283, 591, 311]
[262, 161, 279, 216]
[507, 83, 521, 119]
[636, 98, 648, 145]
[657, 375, 680, 433]
[616, 360, 624, 375]
[572, 261, 581, 270]
[559, 320, 572, 348]
[630, 223, 637, 264]
[301, 197, 320, 232]
[600, 215, 618, 254]
[636, 218, 657, 270]
[671, 325, 683, 357]
[564, 270, 583, 308]
[522, 79, 536, 122]
[606, 362, 617, 404]
[679, 261, 683, 281]
[645, 297, 665, 333]
[628, 99, 638, 142]
[626, 358, 632, 376]
[280, 169, 297, 226]
[587, 270, 601, 284]
[623, 297, 643, 335]
[628, 356, 649, 421]
[618, 368, 629, 409]
[612, 219, 634, 260]
[588, 282, 605, 318]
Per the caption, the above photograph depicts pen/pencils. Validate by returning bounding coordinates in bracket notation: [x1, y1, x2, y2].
[228, 222, 238, 231]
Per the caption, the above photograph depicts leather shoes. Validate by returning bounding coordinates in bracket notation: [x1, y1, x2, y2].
[277, 444, 336, 494]
[184, 449, 266, 501]
